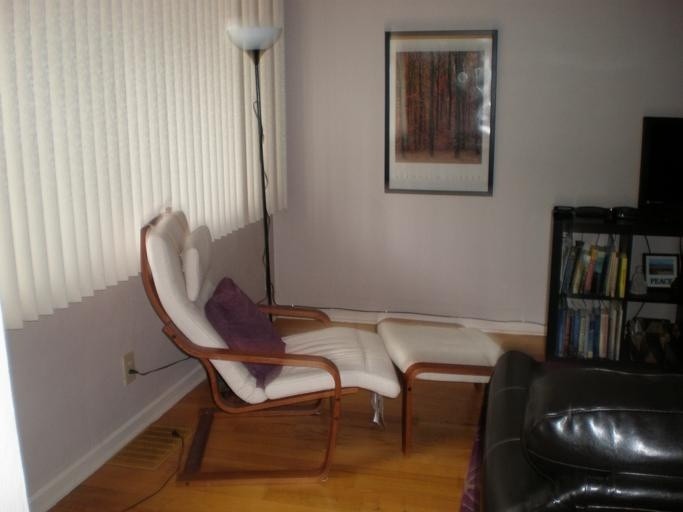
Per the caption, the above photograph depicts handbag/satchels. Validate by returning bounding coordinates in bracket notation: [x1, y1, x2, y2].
[628, 318, 670, 365]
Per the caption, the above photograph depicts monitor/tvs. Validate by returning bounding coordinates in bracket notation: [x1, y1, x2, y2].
[638, 116, 683, 216]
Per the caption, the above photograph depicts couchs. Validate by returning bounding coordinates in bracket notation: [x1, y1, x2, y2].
[461, 347, 682, 512]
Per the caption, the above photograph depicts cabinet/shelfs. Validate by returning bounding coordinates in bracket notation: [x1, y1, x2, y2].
[539, 202, 683, 364]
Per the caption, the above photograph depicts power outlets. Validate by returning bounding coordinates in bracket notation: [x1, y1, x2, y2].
[119, 348, 135, 381]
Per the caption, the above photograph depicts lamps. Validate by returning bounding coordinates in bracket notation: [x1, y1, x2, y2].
[225, 24, 283, 321]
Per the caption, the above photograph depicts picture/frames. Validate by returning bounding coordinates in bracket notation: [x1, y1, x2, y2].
[381, 28, 496, 198]
[642, 253, 680, 293]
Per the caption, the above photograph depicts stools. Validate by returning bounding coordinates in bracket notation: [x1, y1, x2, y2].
[374, 316, 506, 454]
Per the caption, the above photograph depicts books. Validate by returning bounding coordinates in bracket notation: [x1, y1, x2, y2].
[554, 238, 628, 363]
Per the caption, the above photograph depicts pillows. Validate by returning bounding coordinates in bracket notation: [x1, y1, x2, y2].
[204, 277, 286, 389]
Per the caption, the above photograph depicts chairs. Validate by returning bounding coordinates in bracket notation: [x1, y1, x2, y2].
[137, 204, 400, 489]
